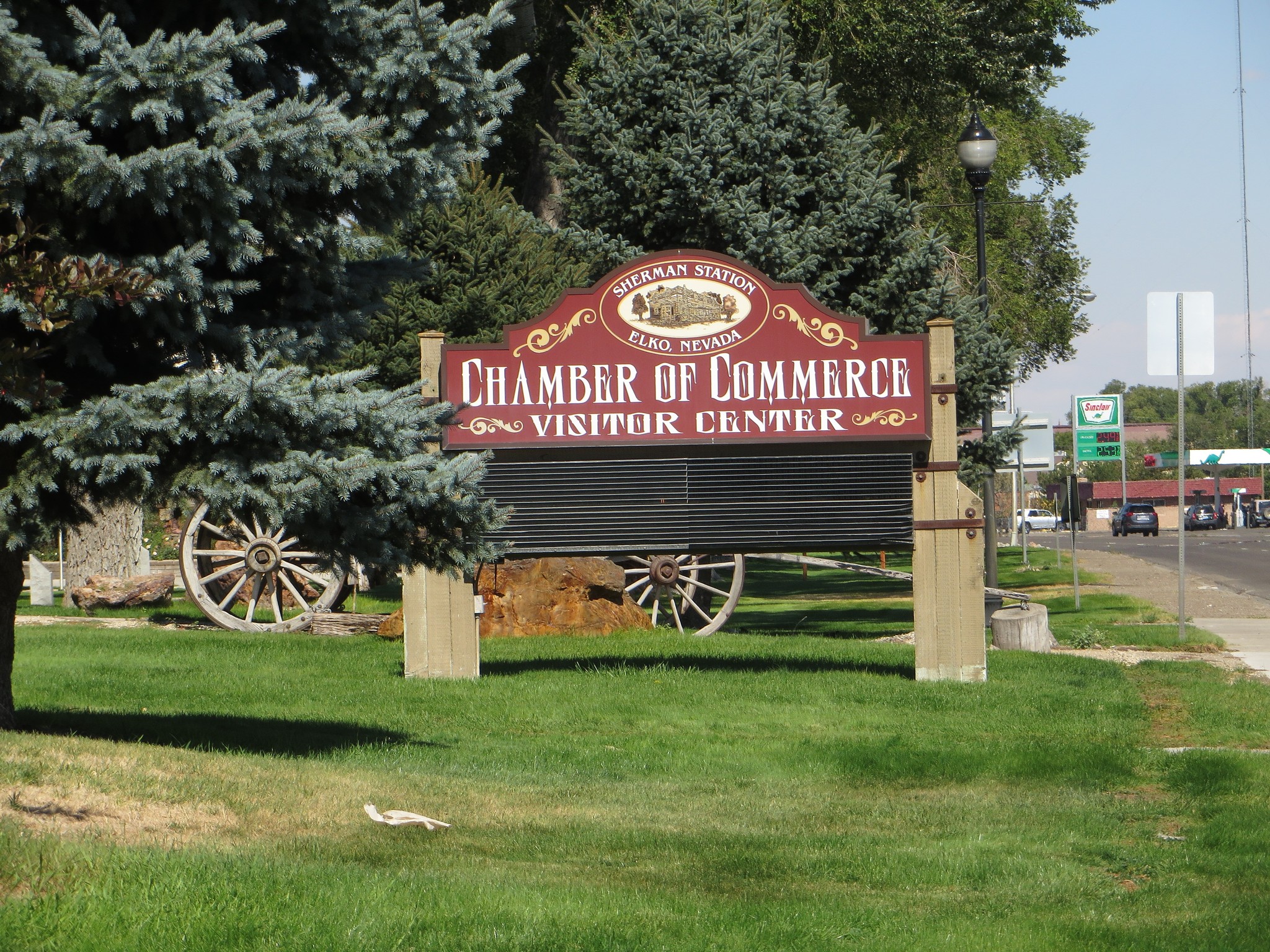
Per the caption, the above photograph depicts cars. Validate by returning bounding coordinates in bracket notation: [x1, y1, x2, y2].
[1183, 504, 1218, 531]
[1007, 508, 1065, 534]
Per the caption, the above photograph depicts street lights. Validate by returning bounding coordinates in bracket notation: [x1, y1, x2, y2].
[954, 111, 1004, 628]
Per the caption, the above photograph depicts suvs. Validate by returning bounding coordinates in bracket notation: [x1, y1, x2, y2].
[1249, 499, 1270, 528]
[1111, 502, 1159, 537]
